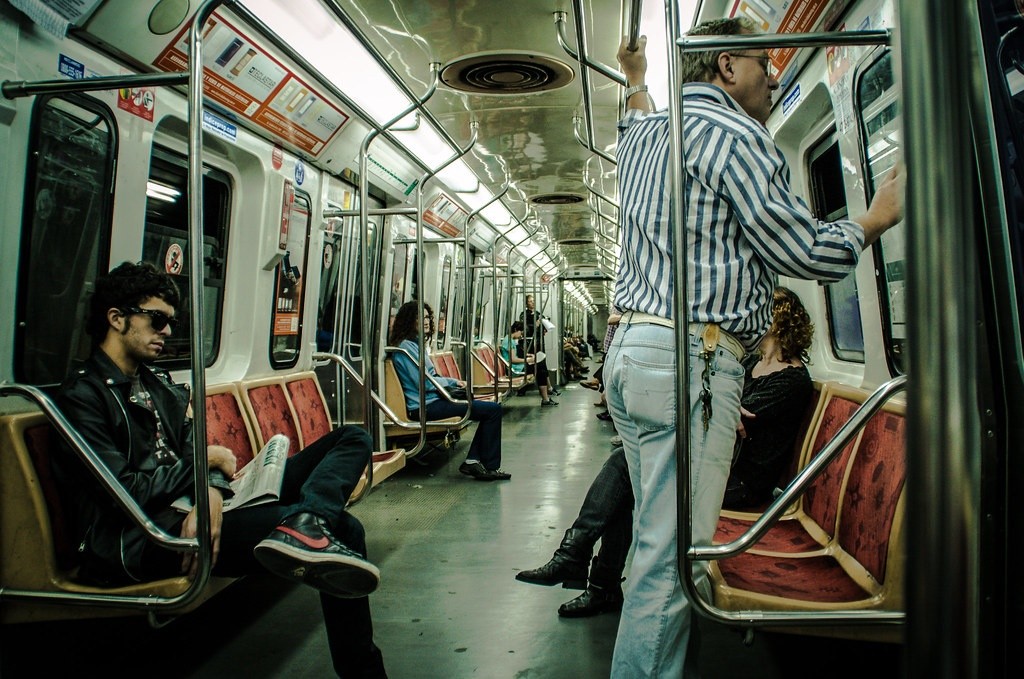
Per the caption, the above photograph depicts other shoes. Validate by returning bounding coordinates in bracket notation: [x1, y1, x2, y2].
[548, 387, 561, 396]
[541, 399, 561, 406]
[594, 401, 607, 407]
[597, 409, 613, 422]
[566, 365, 590, 381]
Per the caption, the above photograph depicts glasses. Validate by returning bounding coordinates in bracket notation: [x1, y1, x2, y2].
[117, 306, 178, 331]
[423, 315, 434, 320]
[730, 54, 773, 77]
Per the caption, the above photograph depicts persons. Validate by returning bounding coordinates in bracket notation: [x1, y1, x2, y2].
[515, 285, 815, 618]
[602, 302, 623, 407]
[517, 294, 550, 396]
[563, 333, 601, 381]
[389, 301, 511, 480]
[602, 16, 907, 679]
[47, 262, 388, 679]
[501, 321, 562, 406]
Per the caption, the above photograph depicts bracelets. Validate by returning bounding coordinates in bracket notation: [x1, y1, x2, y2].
[626, 85, 647, 100]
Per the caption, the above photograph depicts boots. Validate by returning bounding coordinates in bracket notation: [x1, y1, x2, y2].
[558, 557, 624, 618]
[514, 529, 588, 591]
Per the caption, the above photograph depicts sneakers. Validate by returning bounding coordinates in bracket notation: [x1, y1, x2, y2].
[459, 460, 497, 481]
[486, 468, 512, 480]
[253, 511, 380, 598]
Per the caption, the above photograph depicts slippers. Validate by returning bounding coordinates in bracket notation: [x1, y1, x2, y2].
[580, 380, 599, 390]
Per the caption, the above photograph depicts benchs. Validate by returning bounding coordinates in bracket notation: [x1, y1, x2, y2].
[706, 378, 913, 643]
[472, 344, 534, 394]
[0, 370, 406, 628]
[386, 350, 503, 437]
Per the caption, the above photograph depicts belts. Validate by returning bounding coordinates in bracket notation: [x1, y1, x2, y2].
[620, 311, 745, 363]
